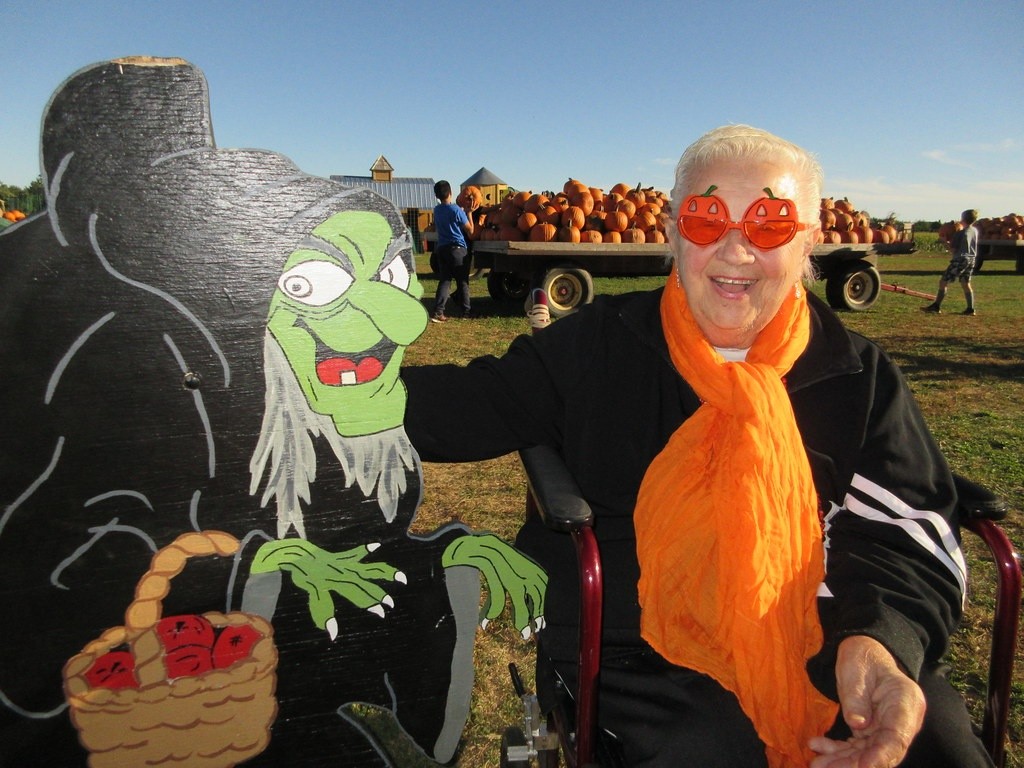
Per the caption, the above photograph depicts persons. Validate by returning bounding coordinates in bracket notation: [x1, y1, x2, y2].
[428, 180, 480, 323]
[399, 122, 1000, 768]
[919, 208, 979, 315]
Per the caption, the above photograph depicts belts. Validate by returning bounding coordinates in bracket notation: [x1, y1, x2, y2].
[449, 246, 461, 248]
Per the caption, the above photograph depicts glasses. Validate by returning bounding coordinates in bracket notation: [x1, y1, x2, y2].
[676, 185, 815, 252]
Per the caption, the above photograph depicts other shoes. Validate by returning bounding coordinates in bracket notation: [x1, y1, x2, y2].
[462, 317, 472, 321]
[432, 314, 446, 323]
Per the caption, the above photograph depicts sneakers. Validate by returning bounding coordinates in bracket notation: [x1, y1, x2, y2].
[962, 308, 975, 315]
[920, 303, 941, 314]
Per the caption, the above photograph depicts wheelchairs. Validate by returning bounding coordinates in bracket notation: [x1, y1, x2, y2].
[506, 471, 1024, 768]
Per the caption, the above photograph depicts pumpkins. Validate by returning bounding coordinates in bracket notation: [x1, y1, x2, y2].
[938, 212, 1024, 251]
[816, 196, 899, 245]
[455, 177, 671, 244]
